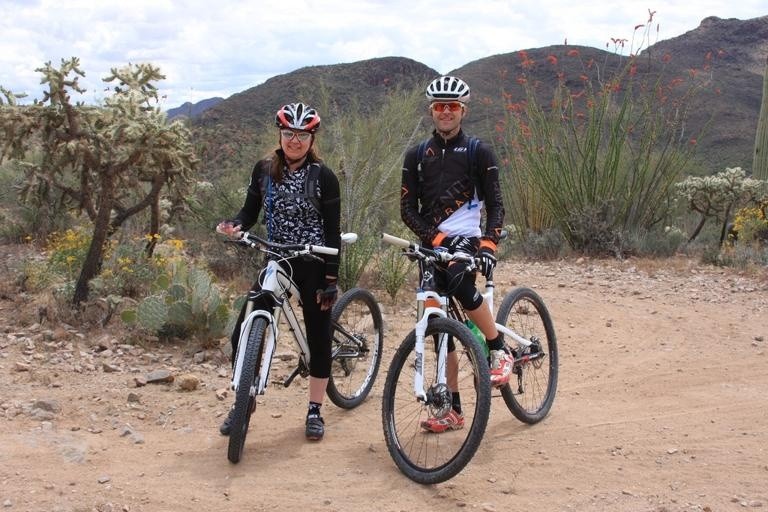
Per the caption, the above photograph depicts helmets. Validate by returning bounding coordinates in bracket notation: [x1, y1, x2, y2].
[275, 102, 320, 132]
[425, 76, 470, 102]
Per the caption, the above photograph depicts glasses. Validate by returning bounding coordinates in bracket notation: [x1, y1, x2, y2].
[280, 129, 311, 141]
[428, 101, 465, 112]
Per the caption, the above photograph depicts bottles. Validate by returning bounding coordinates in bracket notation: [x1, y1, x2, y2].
[463, 318, 490, 360]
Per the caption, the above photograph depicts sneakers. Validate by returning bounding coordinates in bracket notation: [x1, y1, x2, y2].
[421, 409, 464, 433]
[220, 397, 256, 435]
[305, 406, 324, 441]
[488, 347, 514, 386]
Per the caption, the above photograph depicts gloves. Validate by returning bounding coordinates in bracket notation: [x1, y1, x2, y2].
[474, 248, 497, 280]
[442, 235, 476, 254]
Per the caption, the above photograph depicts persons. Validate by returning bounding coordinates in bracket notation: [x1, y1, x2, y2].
[398, 76, 515, 434]
[217, 101, 343, 441]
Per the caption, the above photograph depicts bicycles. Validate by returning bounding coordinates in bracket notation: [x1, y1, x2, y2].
[214, 222, 386, 466]
[376, 231, 559, 487]
